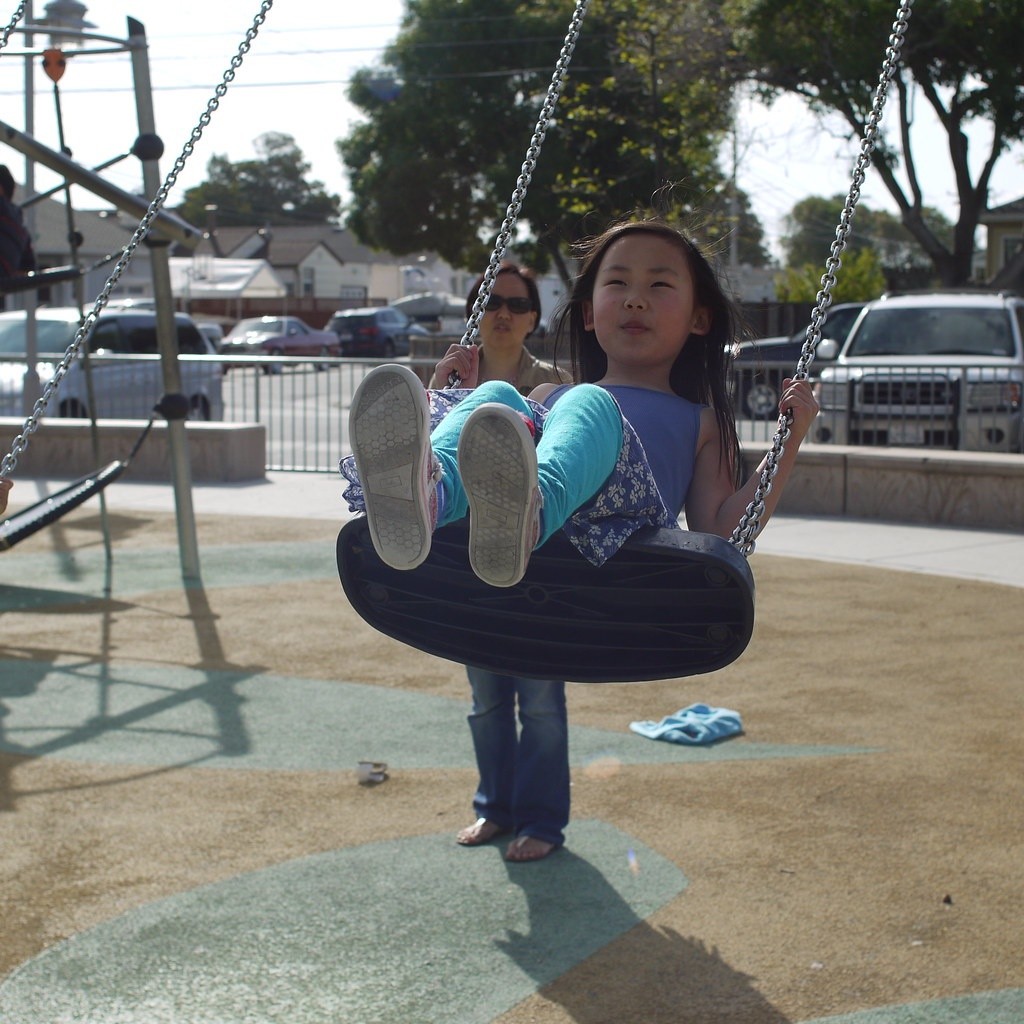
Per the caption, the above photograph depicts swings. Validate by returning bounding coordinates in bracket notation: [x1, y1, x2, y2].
[334, 0, 917, 683]
[0, 0, 282, 556]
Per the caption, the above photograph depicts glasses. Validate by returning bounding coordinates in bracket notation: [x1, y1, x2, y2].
[485, 294, 533, 314]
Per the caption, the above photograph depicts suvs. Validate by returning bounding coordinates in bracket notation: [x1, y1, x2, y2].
[806, 288, 1023, 455]
[319, 307, 431, 369]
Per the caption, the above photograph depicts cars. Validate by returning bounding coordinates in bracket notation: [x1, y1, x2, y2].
[217, 314, 340, 375]
[722, 301, 868, 421]
[0, 294, 226, 426]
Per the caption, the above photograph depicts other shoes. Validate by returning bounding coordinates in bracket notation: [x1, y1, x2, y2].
[457, 402, 545, 588]
[349, 363, 438, 571]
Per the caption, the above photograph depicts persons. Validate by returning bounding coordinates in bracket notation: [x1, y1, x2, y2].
[424, 266, 573, 862]
[347, 219, 820, 590]
